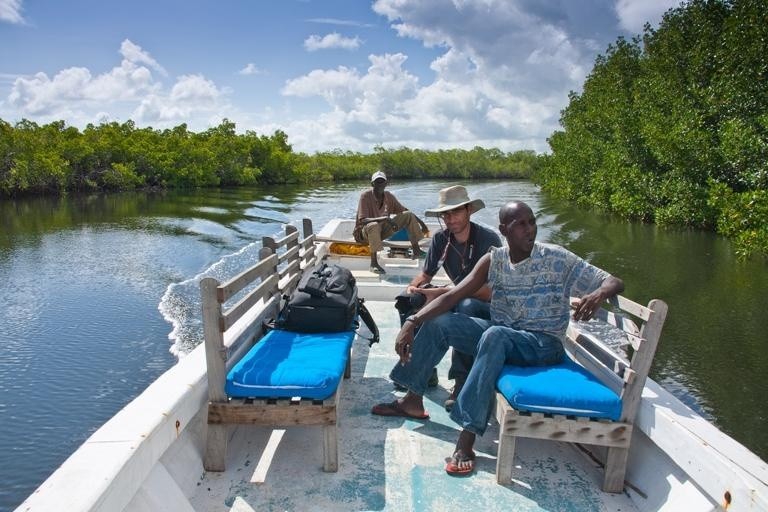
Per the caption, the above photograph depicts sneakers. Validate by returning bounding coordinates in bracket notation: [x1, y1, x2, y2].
[369, 265, 385, 274]
[394, 367, 439, 388]
[413, 252, 426, 259]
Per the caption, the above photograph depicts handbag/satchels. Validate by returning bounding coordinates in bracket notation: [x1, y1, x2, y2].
[286, 264, 358, 333]
[395, 282, 433, 310]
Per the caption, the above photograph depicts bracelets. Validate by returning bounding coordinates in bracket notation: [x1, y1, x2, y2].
[406, 314, 419, 331]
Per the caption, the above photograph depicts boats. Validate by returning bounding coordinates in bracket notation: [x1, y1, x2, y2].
[12, 217, 768, 511]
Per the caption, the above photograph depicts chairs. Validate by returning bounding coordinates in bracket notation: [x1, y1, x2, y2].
[386, 227, 412, 258]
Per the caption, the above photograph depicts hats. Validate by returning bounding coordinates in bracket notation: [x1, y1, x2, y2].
[424, 185, 485, 218]
[371, 170, 387, 183]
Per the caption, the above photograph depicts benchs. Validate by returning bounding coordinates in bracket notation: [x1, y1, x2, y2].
[491, 291, 668, 495]
[197, 217, 359, 473]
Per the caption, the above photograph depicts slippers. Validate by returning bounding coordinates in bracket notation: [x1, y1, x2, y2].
[372, 399, 428, 419]
[446, 450, 475, 474]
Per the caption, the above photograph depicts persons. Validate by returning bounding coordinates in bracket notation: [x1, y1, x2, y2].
[353, 171, 430, 274]
[372, 199, 626, 476]
[395, 185, 504, 414]
